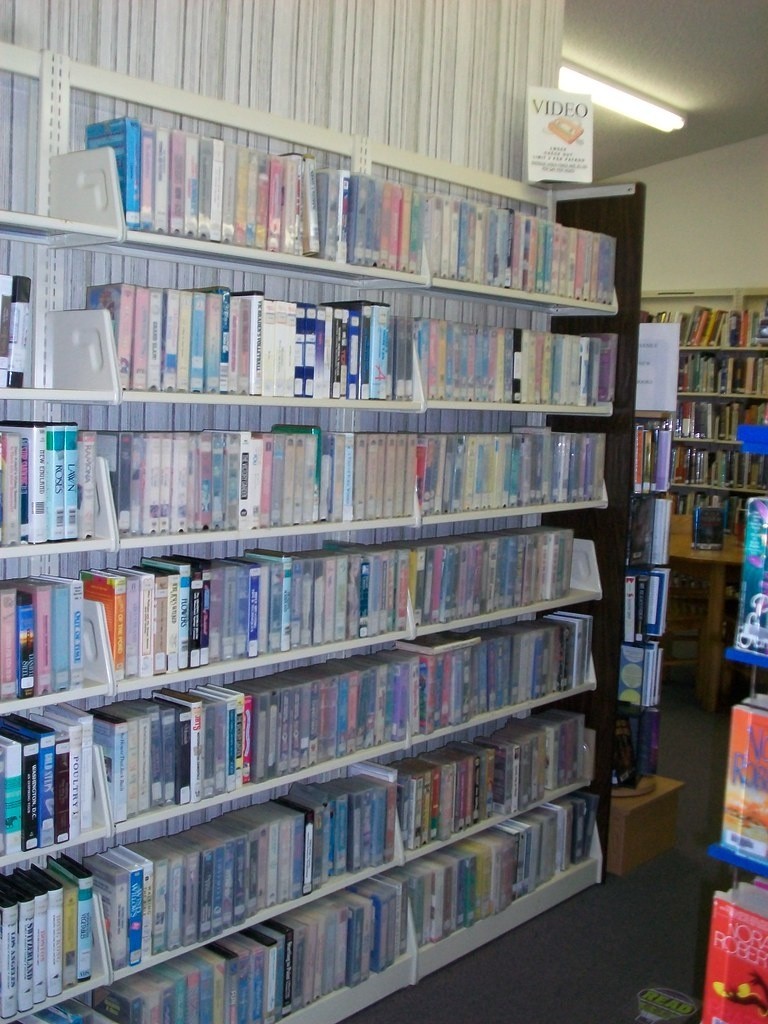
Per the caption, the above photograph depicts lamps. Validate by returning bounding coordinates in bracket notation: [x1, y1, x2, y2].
[558, 61, 688, 134]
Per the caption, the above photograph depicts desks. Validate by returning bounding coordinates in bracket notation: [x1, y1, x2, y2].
[664, 511, 754, 706]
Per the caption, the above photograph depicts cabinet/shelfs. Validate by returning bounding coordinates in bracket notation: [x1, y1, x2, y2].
[0, 44, 648, 1024]
[637, 289, 767, 539]
[677, 493, 768, 1023]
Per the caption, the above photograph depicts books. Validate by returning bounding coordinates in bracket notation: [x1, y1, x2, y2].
[521, 87, 594, 186]
[638, 306, 768, 662]
[614, 423, 673, 789]
[701, 496, 768, 1024]
[0, 118, 616, 1024]
